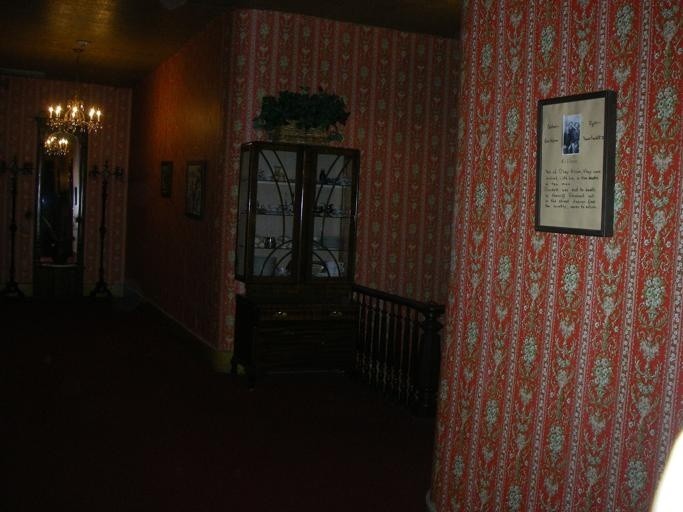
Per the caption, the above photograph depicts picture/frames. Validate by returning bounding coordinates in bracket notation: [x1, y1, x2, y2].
[161, 160, 172, 196]
[184, 160, 206, 219]
[537, 90, 614, 237]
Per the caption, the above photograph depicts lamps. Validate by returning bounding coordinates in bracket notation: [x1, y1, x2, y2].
[45, 48, 103, 158]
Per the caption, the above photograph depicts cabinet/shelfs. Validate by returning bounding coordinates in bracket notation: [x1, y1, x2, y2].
[234, 142, 361, 390]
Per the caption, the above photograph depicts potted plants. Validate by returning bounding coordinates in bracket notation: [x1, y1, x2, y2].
[252, 84, 350, 145]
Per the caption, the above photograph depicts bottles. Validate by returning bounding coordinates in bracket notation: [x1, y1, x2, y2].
[319, 168, 326, 184]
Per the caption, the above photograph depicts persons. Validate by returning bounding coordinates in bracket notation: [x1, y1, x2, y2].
[567, 122, 580, 153]
